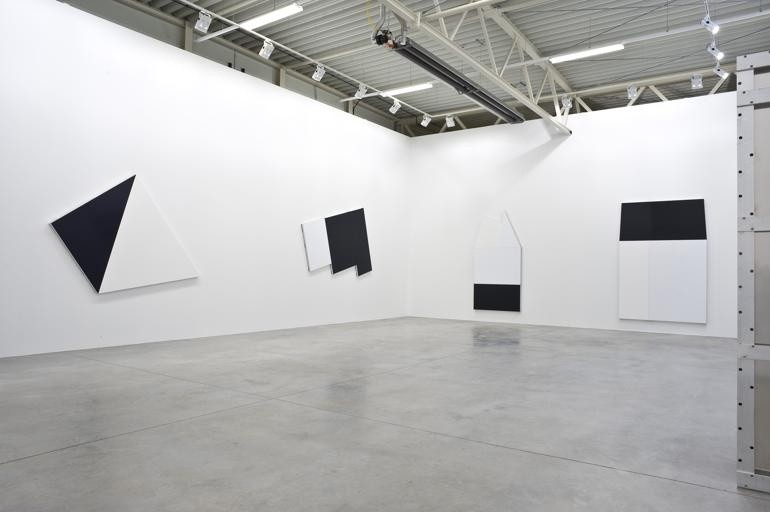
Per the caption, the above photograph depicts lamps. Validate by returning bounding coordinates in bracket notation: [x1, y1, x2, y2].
[311, 66, 327, 83]
[258, 39, 275, 61]
[194, 9, 214, 36]
[560, 94, 575, 110]
[691, 16, 730, 91]
[353, 84, 456, 129]
[626, 85, 639, 100]
